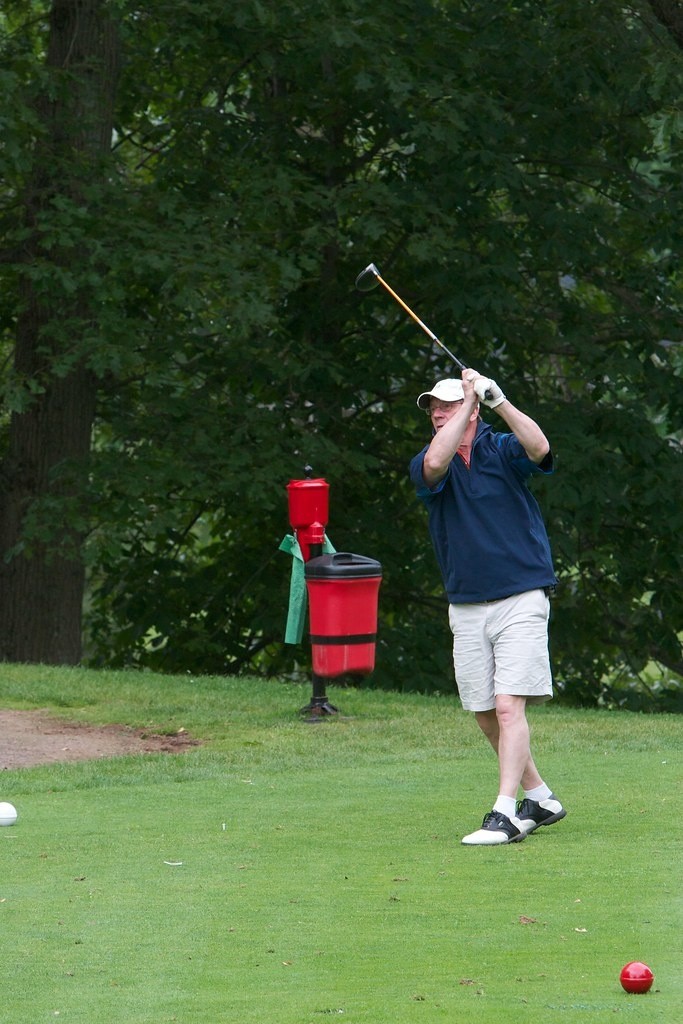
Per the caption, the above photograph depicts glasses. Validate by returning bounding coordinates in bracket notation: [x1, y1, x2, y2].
[426, 402, 463, 415]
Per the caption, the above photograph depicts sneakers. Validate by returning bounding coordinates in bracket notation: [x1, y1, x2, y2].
[515, 792, 567, 835]
[461, 809, 527, 845]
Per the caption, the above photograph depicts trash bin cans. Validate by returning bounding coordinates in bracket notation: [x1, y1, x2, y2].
[303, 552, 383, 678]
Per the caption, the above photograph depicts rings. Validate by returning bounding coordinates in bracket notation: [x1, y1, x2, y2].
[467, 375, 474, 381]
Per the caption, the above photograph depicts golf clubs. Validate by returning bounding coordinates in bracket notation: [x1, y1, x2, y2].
[354, 262, 495, 401]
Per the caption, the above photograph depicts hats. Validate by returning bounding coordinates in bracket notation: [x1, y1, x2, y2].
[417, 378, 479, 410]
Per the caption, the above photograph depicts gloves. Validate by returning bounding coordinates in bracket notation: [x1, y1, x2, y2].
[462, 368, 506, 410]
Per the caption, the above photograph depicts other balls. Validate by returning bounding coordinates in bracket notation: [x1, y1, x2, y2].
[619, 961, 654, 994]
[0, 801, 18, 827]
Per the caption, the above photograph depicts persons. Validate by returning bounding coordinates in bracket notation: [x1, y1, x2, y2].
[409, 368, 567, 845]
